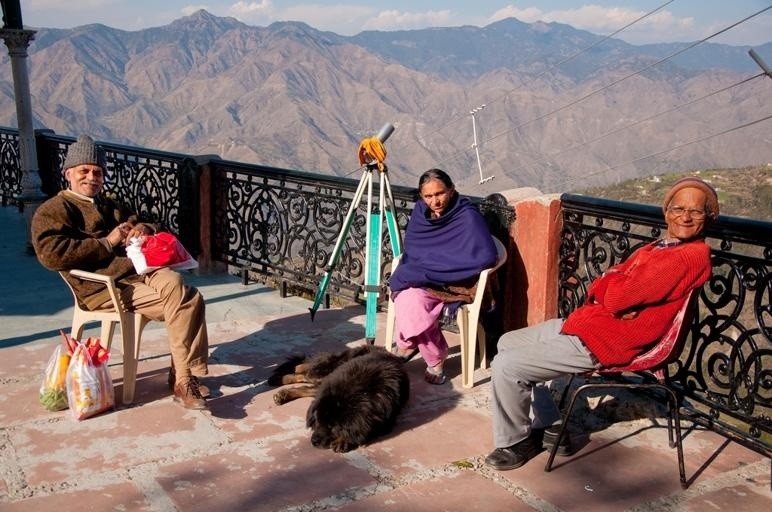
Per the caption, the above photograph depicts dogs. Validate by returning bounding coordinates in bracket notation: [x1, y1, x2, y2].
[267, 343, 411, 454]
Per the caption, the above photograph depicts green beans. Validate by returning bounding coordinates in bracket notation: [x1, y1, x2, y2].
[39, 389, 68, 412]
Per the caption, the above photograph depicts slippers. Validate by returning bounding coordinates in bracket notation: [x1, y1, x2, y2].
[425, 365, 448, 384]
[392, 345, 419, 363]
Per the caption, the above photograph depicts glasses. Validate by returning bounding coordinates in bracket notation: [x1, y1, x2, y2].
[668, 206, 708, 220]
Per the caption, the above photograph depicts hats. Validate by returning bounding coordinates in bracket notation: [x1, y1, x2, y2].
[663, 176, 719, 215]
[63, 134, 107, 175]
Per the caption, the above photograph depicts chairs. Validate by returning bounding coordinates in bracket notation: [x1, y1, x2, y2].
[383, 229, 509, 390]
[544, 285, 700, 490]
[58, 267, 151, 406]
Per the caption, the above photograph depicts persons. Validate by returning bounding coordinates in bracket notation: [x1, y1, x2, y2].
[30, 133, 213, 411]
[484, 176, 719, 472]
[386, 168, 497, 386]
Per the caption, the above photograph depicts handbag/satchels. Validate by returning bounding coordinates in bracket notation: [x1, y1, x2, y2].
[125, 231, 199, 278]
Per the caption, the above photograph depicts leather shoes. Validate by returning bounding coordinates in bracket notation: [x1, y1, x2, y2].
[168, 368, 211, 398]
[173, 378, 208, 410]
[485, 433, 543, 471]
[533, 427, 573, 457]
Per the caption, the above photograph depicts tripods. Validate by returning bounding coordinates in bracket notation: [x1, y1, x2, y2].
[308, 161, 405, 345]
